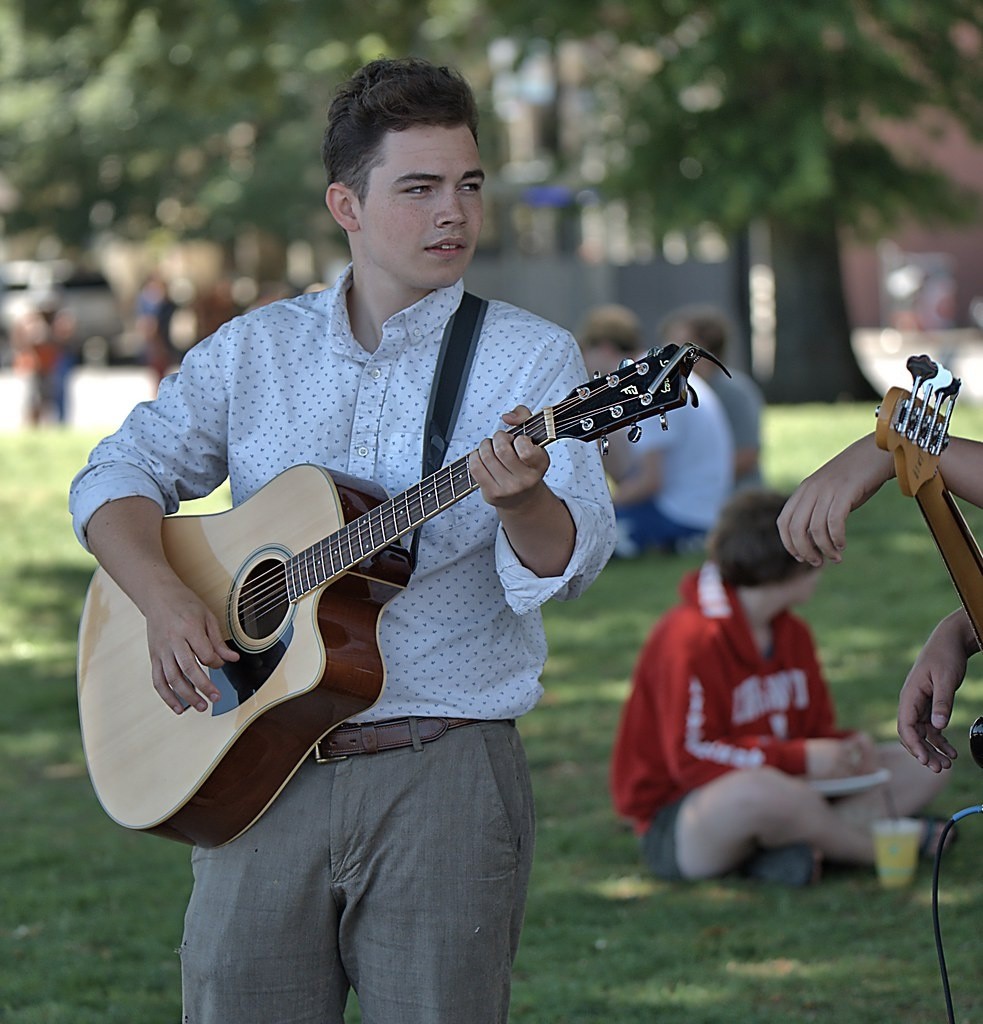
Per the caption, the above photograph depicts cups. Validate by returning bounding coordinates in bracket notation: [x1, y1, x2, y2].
[872, 821, 923, 890]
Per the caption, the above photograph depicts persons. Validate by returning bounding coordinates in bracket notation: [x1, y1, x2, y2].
[66, 50, 618, 1023]
[613, 491, 961, 892]
[16, 268, 235, 426]
[777, 430, 983, 774]
[576, 298, 769, 559]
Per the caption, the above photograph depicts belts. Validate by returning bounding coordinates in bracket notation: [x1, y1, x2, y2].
[314, 714, 513, 765]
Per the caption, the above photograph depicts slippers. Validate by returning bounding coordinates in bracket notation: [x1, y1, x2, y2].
[876, 812, 958, 863]
[743, 840, 817, 892]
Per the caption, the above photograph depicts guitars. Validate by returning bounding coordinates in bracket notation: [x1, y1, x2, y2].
[873, 351, 982, 779]
[75, 340, 730, 845]
